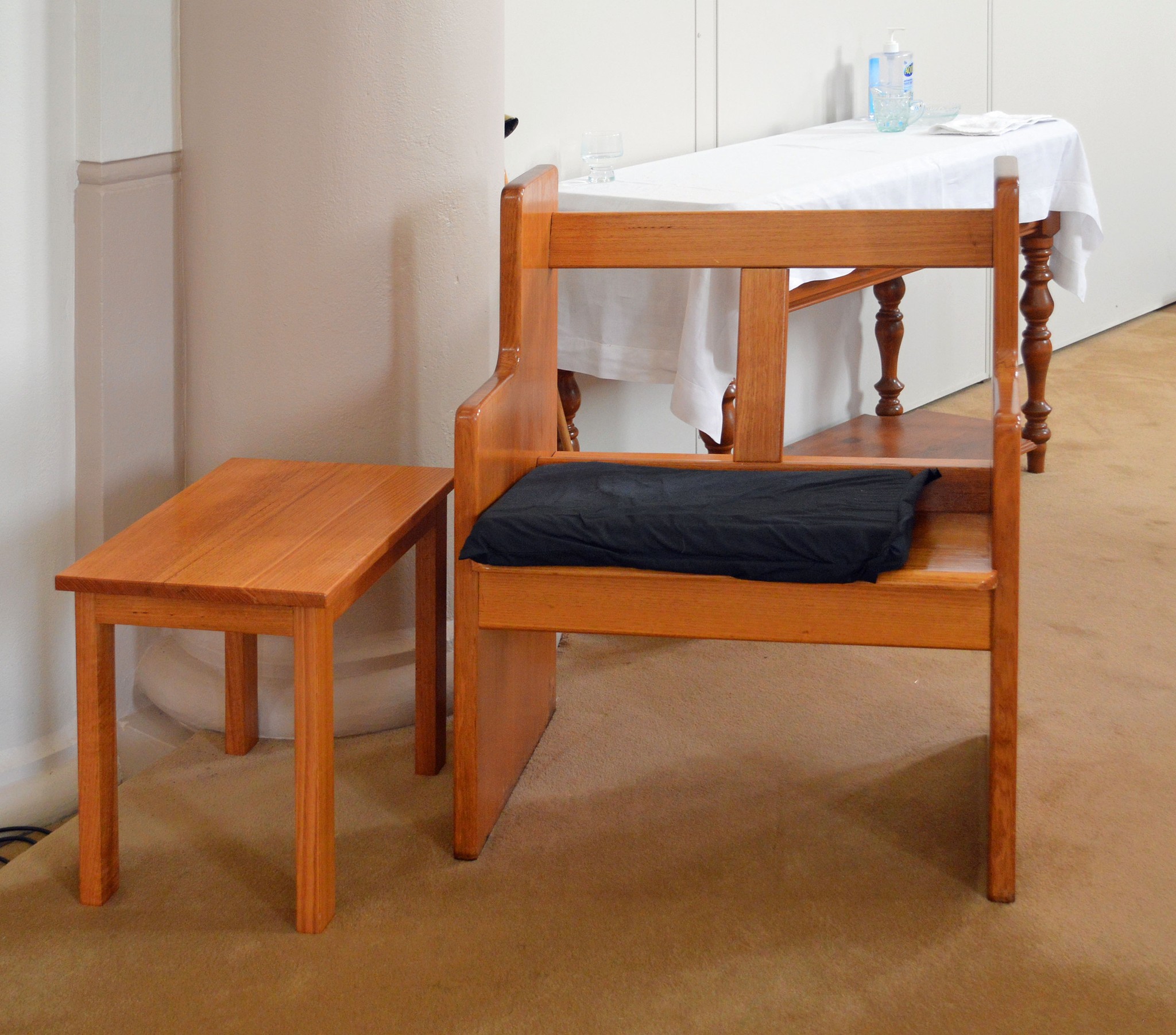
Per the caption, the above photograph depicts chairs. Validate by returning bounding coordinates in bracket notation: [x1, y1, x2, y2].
[452, 155, 1021, 904]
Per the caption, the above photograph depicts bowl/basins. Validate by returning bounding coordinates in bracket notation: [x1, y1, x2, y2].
[908, 101, 958, 124]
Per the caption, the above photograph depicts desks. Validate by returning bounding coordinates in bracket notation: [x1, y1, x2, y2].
[559, 112, 1074, 475]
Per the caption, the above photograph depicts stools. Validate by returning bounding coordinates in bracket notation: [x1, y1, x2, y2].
[54, 459, 455, 933]
